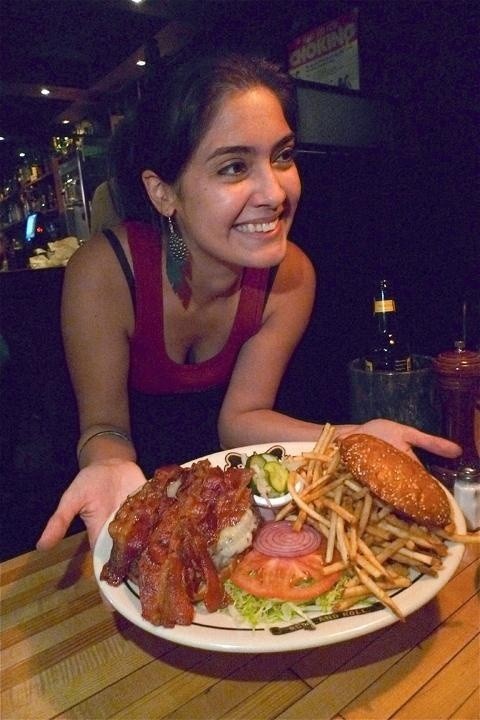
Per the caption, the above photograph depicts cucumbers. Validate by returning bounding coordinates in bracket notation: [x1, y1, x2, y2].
[245, 452, 290, 496]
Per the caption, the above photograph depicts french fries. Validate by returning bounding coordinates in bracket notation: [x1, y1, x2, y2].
[275, 424, 479, 623]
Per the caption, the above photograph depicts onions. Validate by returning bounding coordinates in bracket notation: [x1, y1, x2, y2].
[251, 520, 322, 558]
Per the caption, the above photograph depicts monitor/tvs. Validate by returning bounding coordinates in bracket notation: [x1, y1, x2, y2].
[24, 212, 39, 243]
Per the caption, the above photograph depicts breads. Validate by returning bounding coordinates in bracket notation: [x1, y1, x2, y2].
[339, 430, 450, 530]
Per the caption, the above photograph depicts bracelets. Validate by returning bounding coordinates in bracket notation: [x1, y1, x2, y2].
[77, 424, 131, 464]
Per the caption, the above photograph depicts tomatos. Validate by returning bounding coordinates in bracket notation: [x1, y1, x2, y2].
[228, 536, 345, 602]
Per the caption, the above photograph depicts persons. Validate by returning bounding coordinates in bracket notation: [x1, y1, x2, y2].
[36, 47, 461, 551]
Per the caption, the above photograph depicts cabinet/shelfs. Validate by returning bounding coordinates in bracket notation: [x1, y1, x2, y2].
[1, 155, 64, 236]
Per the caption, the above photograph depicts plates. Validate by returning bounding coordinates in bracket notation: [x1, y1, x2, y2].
[91, 440, 468, 654]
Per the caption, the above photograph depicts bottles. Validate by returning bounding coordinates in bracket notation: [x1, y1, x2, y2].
[453, 464, 480, 534]
[363, 277, 417, 425]
